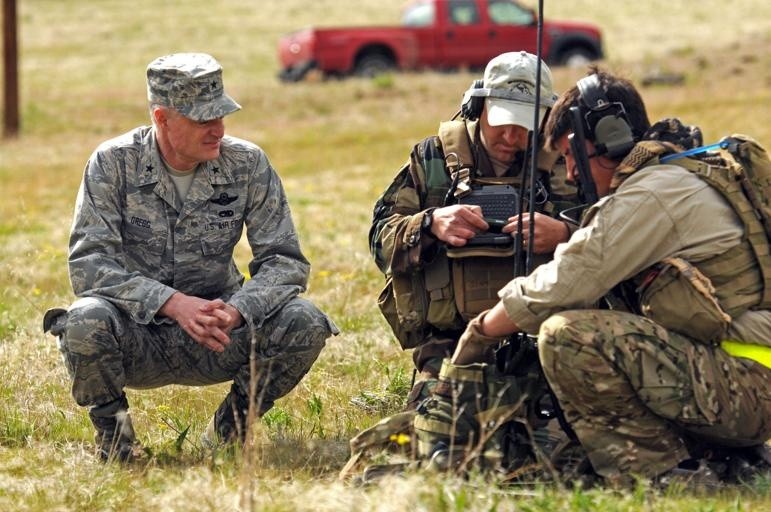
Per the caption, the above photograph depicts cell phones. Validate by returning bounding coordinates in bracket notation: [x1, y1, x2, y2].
[481, 218, 513, 235]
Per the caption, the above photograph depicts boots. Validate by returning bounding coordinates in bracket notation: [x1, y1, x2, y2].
[89, 392, 134, 463]
[200, 385, 274, 456]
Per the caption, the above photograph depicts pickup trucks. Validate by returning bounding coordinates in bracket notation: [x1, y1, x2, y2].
[275, 0, 606, 82]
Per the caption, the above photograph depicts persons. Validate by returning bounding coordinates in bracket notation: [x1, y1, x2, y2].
[453, 64, 770, 496]
[367, 52, 583, 378]
[42, 51, 340, 463]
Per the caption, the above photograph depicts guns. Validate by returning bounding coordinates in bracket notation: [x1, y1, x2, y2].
[561, 105, 644, 314]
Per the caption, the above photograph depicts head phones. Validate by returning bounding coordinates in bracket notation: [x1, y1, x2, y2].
[576, 73, 641, 161]
[460, 80, 561, 121]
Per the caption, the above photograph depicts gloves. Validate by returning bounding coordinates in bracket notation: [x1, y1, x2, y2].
[433, 308, 509, 414]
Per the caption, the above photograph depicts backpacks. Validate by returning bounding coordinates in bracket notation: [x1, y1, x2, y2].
[636, 132, 770, 345]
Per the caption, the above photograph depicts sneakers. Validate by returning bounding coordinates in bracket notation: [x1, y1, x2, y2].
[651, 459, 724, 494]
[706, 442, 771, 485]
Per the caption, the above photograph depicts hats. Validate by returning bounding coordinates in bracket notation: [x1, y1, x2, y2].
[146, 53, 243, 123]
[483, 50, 553, 133]
